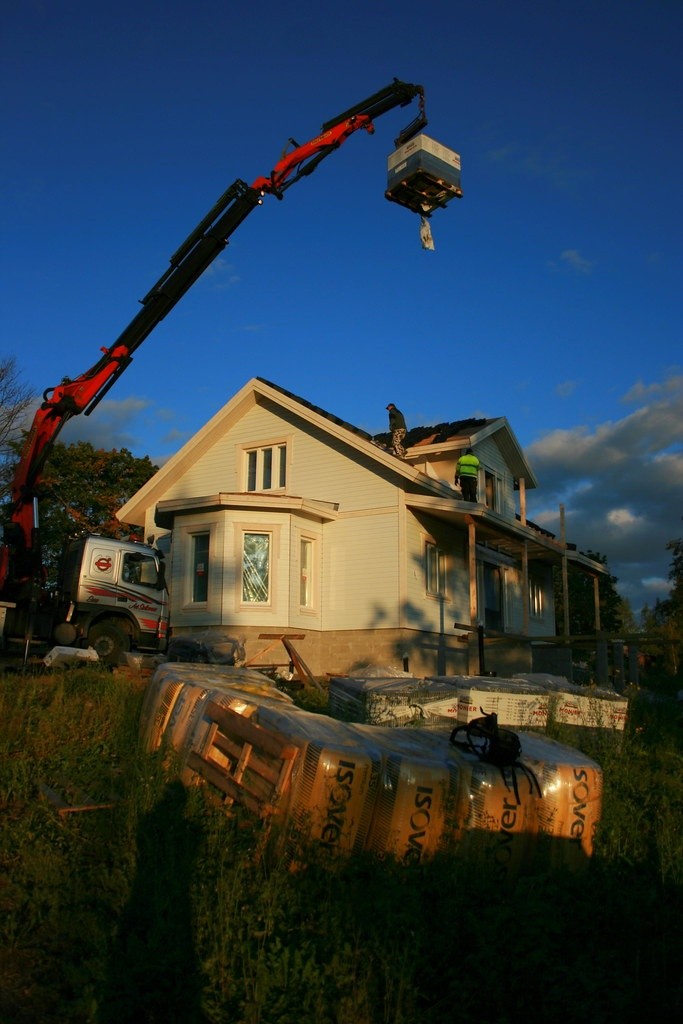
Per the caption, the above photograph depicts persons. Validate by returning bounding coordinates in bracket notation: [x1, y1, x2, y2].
[455, 449, 481, 503]
[386, 403, 408, 457]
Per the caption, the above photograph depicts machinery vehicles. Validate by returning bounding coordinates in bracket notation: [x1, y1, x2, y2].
[0, 75, 465, 673]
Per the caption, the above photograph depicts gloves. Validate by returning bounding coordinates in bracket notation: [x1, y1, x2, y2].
[454, 475, 459, 487]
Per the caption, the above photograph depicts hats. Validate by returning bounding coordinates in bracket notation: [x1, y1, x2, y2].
[386, 403, 395, 410]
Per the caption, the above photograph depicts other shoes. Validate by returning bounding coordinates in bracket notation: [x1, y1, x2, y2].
[401, 450, 408, 457]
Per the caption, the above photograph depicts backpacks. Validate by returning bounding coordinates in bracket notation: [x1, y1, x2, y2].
[450, 709, 523, 768]
